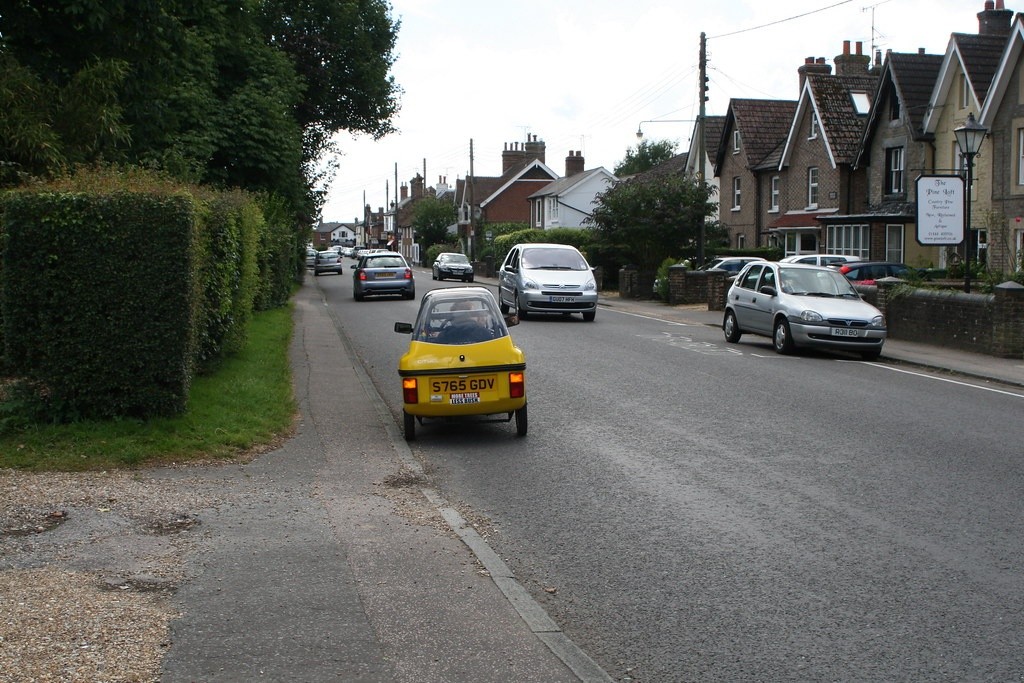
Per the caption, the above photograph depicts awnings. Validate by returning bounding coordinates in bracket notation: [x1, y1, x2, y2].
[768, 207, 839, 240]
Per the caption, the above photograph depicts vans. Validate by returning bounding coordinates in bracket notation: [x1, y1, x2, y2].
[728, 253, 860, 281]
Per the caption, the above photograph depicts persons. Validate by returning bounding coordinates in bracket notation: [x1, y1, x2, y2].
[437, 302, 493, 343]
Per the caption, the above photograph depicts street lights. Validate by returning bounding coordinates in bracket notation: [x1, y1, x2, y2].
[955, 111, 989, 292]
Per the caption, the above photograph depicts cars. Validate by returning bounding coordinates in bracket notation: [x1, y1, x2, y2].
[837, 260, 949, 283]
[394, 285, 527, 443]
[351, 253, 415, 301]
[721, 261, 887, 356]
[433, 252, 475, 283]
[499, 243, 598, 322]
[307, 243, 390, 275]
[696, 256, 768, 280]
